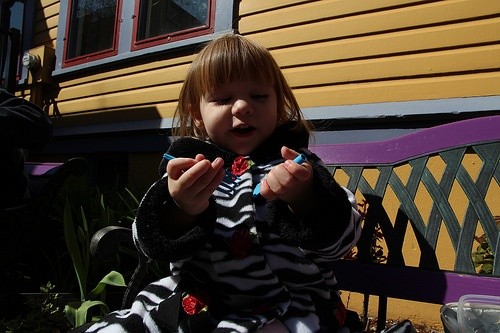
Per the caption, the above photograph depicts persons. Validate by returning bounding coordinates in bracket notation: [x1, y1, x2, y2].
[132, 34, 360, 333]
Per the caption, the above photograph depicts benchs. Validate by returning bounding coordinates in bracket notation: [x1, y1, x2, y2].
[90, 114, 500, 330]
